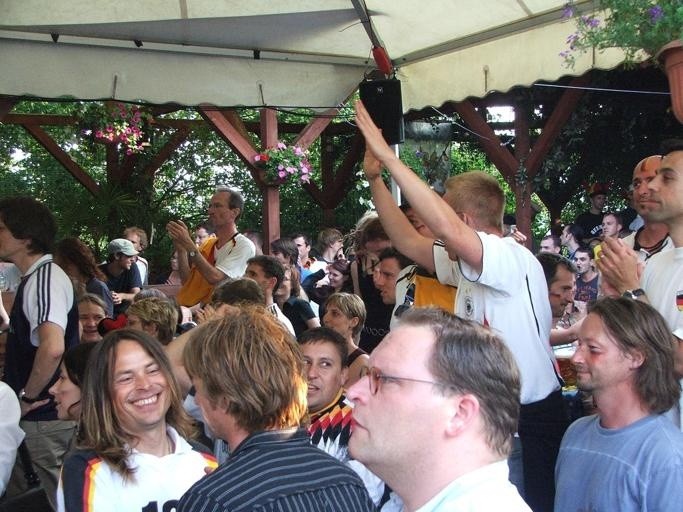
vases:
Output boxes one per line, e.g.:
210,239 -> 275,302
258,166 -> 285,186
95,130 -> 122,146
655,39 -> 683,123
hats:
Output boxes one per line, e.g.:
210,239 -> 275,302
106,238 -> 139,257
587,183 -> 608,197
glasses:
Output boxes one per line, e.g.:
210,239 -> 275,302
358,366 -> 467,395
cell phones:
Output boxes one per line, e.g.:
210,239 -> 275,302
509,225 -> 517,234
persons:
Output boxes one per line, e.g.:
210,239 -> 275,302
1,99 -> 682,511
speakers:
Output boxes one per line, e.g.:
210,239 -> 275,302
358,70 -> 406,145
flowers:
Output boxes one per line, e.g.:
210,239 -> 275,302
254,142 -> 315,186
94,101 -> 151,156
558,0 -> 683,68
